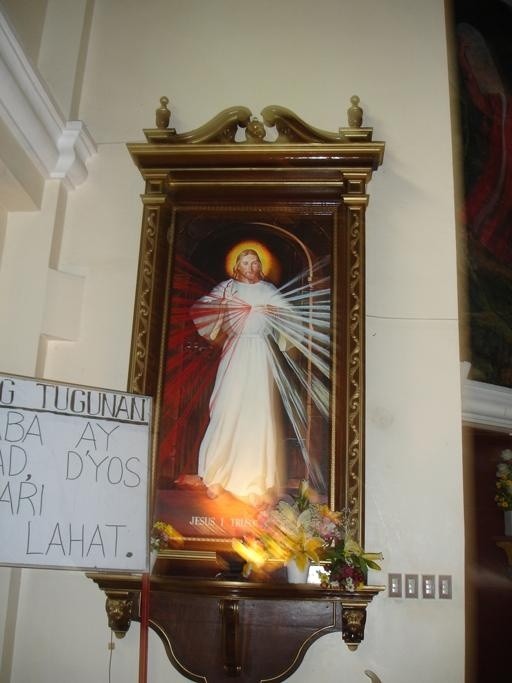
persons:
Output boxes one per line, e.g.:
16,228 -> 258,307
190,250 -> 307,501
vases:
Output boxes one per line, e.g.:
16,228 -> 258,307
149,548 -> 160,577
284,555 -> 311,584
504,510 -> 512,537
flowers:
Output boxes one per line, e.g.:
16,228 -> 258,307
229,479 -> 343,577
148,519 -> 185,550
317,543 -> 384,593
494,448 -> 512,510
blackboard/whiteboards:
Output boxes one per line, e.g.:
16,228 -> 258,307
0,373 -> 156,575
